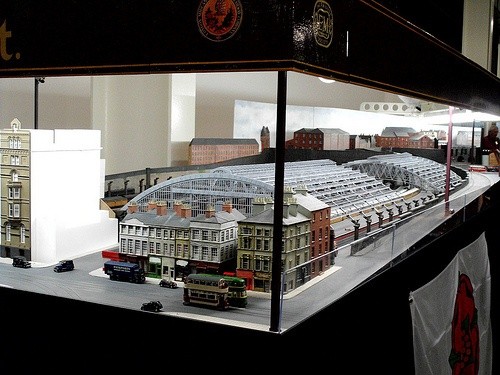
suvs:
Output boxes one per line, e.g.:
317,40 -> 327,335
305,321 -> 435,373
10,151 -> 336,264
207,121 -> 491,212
12,256 -> 31,268
54,260 -> 74,273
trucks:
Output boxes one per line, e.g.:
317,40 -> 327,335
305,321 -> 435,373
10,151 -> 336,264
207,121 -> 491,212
104,261 -> 145,283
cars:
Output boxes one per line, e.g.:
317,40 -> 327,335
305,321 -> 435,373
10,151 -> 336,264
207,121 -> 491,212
142,301 -> 163,311
159,279 -> 178,288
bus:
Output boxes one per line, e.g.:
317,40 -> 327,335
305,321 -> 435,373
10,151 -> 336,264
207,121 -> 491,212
468,165 -> 486,172
183,274 -> 247,310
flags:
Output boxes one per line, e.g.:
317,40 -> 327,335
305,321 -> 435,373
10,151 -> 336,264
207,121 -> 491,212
408,231 -> 492,374
